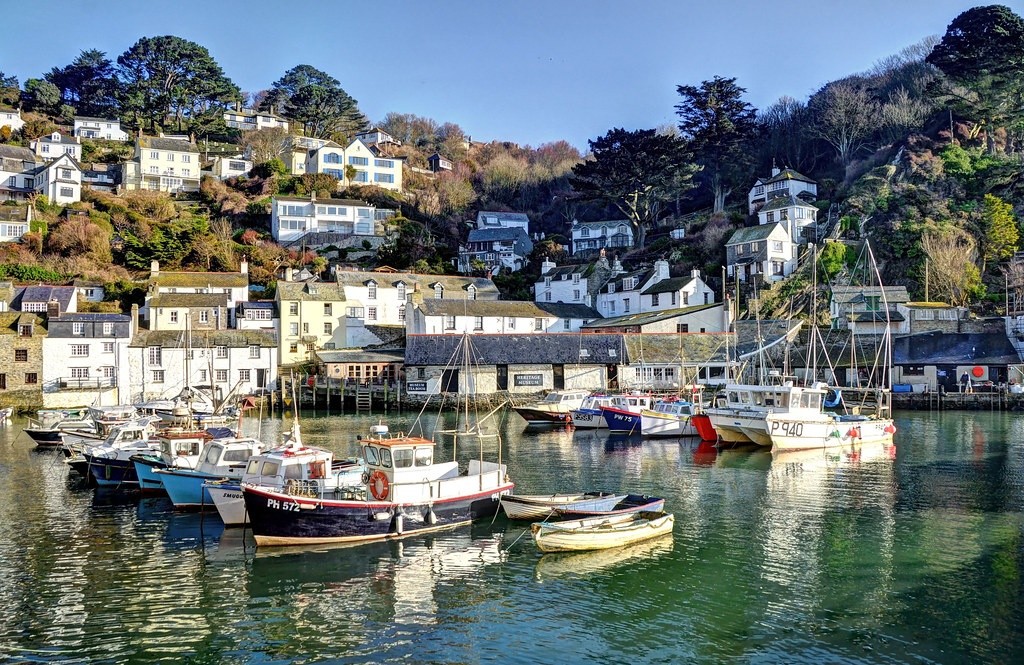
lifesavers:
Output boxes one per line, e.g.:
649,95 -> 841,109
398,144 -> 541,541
369,471 -> 389,501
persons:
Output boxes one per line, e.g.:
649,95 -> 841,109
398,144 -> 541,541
960,371 -> 972,394
997,374 -> 1006,393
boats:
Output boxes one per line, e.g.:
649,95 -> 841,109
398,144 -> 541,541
510,239 -> 898,452
497,488 -> 676,552
19,313 -> 515,548
532,531 -> 676,586
